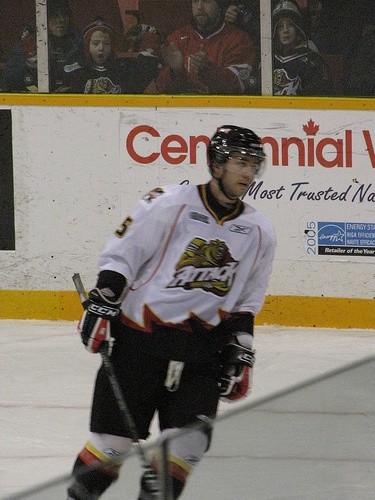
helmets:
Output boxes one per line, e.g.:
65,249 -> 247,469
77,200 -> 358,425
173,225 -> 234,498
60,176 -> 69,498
205,125 -> 266,176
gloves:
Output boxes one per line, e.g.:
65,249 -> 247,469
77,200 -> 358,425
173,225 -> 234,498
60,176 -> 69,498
222,343 -> 256,401
77,287 -> 122,357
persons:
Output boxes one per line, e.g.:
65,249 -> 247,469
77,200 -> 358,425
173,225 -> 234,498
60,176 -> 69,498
66,124 -> 277,500
0,0 -> 375,98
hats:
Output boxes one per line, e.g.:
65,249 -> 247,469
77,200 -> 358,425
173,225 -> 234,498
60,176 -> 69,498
80,17 -> 117,51
20,30 -> 40,60
273,0 -> 305,37
